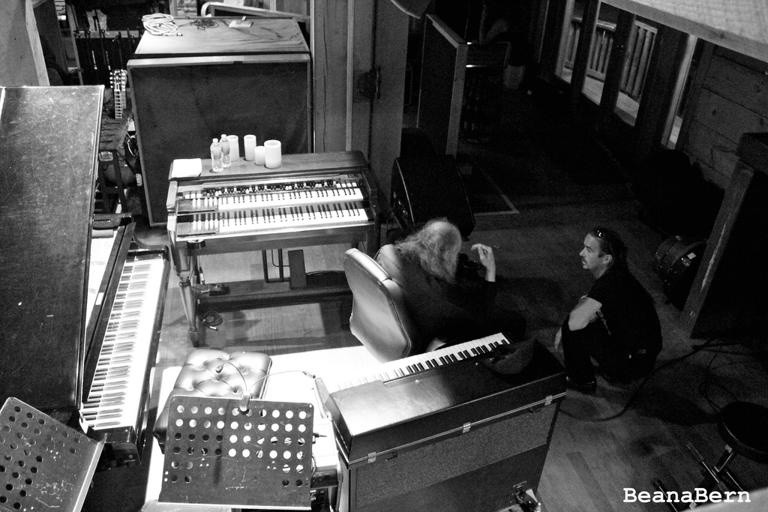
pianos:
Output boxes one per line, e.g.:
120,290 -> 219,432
322,331 -> 511,395
78,252 -> 168,430
177,170 -> 374,238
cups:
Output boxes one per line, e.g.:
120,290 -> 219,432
243,134 -> 256,161
264,140 -> 283,169
254,146 -> 265,165
228,136 -> 240,163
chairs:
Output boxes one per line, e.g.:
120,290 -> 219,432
386,153 -> 478,242
342,245 -> 446,364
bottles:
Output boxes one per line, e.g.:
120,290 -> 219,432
220,134 -> 231,168
210,138 -> 223,171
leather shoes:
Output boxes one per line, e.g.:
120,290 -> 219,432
573,379 -> 597,393
605,354 -> 651,383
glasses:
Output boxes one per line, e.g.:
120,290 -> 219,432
593,226 -> 608,239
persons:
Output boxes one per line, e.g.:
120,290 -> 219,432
554,226 -> 663,395
374,217 -> 527,343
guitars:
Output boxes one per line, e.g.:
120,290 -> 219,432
101,69 -> 138,186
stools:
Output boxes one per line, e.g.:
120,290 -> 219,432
651,399 -> 767,512
149,344 -> 274,460
